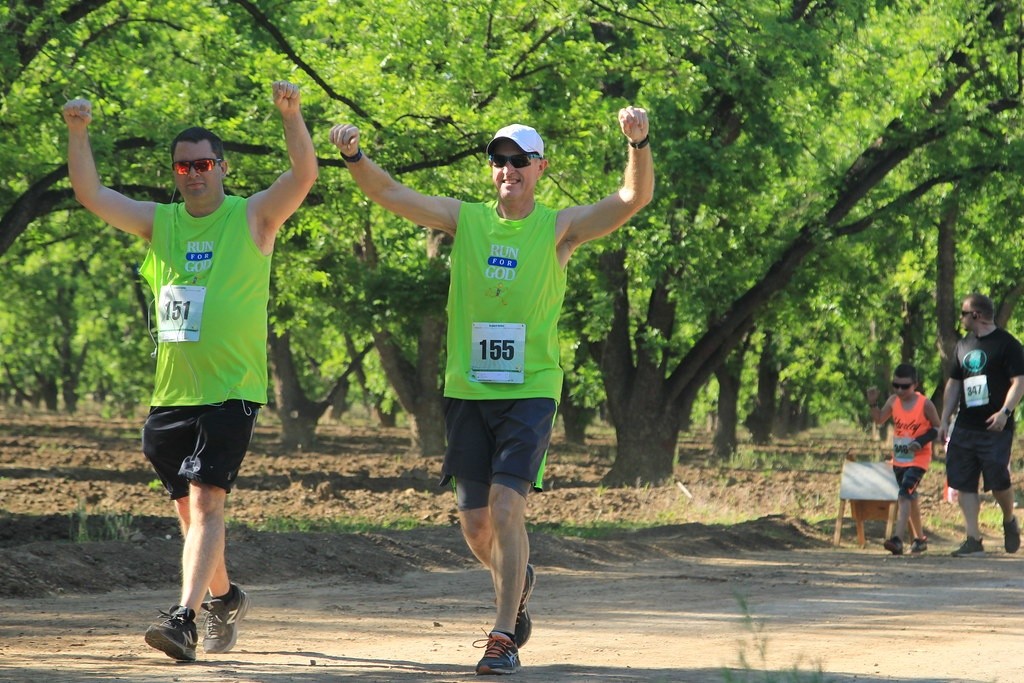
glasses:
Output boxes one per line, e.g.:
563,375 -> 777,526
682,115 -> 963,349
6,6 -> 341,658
174,157 -> 220,175
487,154 -> 540,168
961,310 -> 980,316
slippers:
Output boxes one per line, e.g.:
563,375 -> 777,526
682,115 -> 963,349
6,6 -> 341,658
890,380 -> 915,390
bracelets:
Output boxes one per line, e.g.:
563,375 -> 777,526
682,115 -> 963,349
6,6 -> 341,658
870,403 -> 878,407
629,134 -> 649,149
340,147 -> 362,162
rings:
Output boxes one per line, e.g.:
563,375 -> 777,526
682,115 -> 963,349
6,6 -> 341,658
998,426 -> 1001,430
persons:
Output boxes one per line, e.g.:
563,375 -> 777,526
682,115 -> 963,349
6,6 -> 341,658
329,105 -> 654,673
868,364 -> 941,555
65,82 -> 318,662
938,293 -> 1024,557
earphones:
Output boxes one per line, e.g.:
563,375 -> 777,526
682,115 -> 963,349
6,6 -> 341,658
973,316 -> 977,320
152,348 -> 158,358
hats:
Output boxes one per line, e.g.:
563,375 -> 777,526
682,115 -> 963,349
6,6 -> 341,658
487,122 -> 545,156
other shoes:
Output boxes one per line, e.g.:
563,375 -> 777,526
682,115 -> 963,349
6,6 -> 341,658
882,537 -> 903,555
907,535 -> 928,555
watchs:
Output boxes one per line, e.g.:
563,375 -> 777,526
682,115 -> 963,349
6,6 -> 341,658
1001,407 -> 1012,418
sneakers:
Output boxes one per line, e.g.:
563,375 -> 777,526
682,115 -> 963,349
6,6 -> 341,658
471,626 -> 524,674
951,535 -> 986,557
144,604 -> 197,664
1003,515 -> 1020,553
200,582 -> 249,654
515,563 -> 536,647
